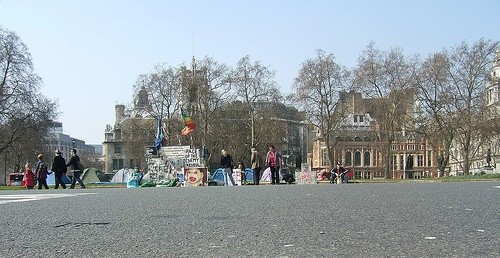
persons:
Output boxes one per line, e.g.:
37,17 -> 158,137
238,161 -> 246,185
219,149 -> 238,187
330,164 -> 348,184
33,154 -> 52,190
487,148 -> 491,167
66,149 -> 85,189
265,145 -> 282,185
138,86 -> 148,105
50,149 -> 67,189
24,163 -> 35,190
284,171 -> 296,185
251,148 -> 263,185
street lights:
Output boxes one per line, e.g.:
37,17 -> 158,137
72,139 -> 76,150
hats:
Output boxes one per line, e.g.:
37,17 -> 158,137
55,150 -> 62,154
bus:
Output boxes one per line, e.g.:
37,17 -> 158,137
9,171 -> 54,186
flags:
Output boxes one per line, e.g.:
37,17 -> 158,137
180,107 -> 196,137
152,116 -> 163,151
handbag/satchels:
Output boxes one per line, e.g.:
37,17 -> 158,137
77,160 -> 84,171
